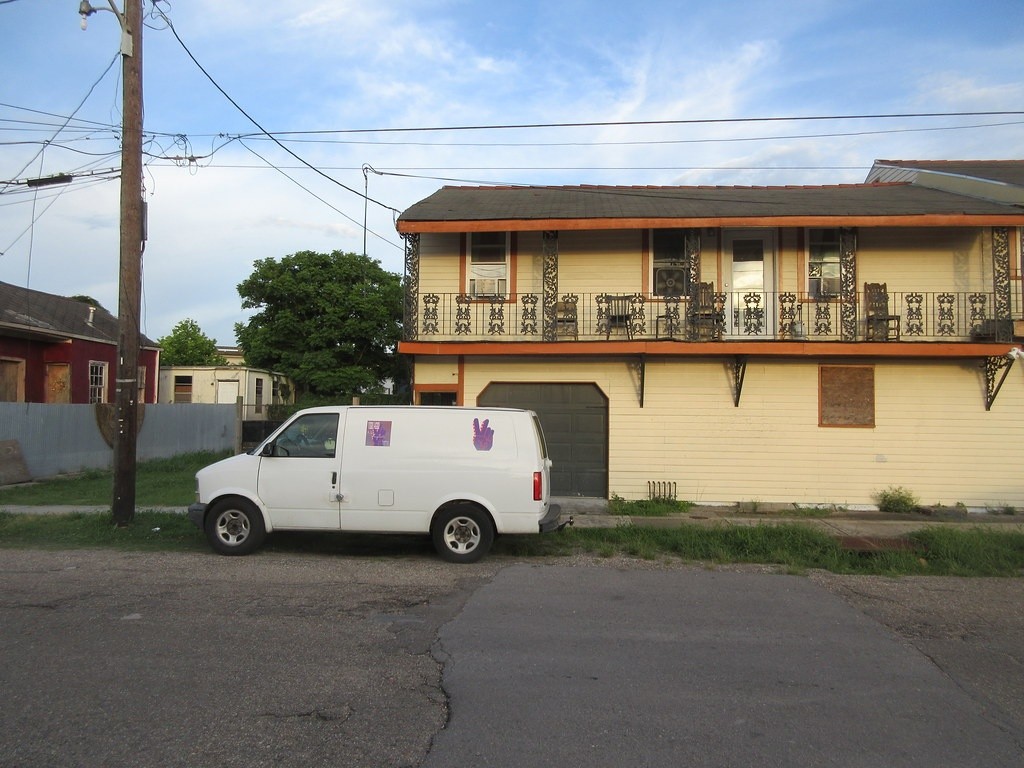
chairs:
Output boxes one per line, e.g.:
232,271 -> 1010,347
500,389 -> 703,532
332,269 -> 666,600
864,282 -> 900,341
552,302 -> 578,341
605,295 -> 633,340
689,281 -> 723,341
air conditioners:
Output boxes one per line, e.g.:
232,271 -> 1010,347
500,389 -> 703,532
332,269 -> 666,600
821,277 -> 841,296
475,278 -> 498,297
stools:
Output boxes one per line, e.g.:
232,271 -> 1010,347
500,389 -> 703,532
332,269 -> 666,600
656,315 -> 677,339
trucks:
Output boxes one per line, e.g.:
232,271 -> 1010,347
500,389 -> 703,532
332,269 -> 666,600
186,405 -> 553,565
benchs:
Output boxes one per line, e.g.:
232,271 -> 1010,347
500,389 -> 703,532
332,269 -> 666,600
969,319 -> 1016,341
